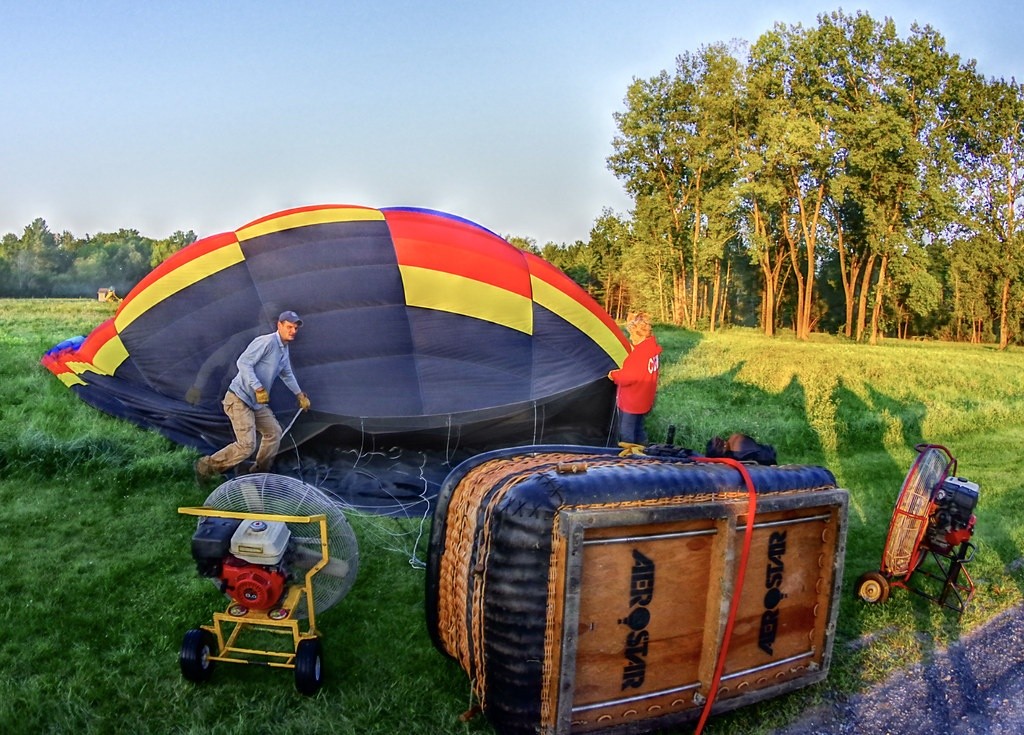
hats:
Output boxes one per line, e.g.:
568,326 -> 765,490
279,312 -> 303,328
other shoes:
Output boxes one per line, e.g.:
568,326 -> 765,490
198,458 -> 211,478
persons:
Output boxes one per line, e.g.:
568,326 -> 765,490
608,311 -> 663,446
198,311 -> 311,480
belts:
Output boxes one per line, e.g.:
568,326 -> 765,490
228,387 -> 236,394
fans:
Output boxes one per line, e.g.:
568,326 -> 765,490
176,472 -> 359,697
857,444 -> 984,623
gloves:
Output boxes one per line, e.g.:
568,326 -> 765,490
256,388 -> 268,403
298,395 -> 311,413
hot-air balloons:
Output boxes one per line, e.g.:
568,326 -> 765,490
41,204 -> 850,735
